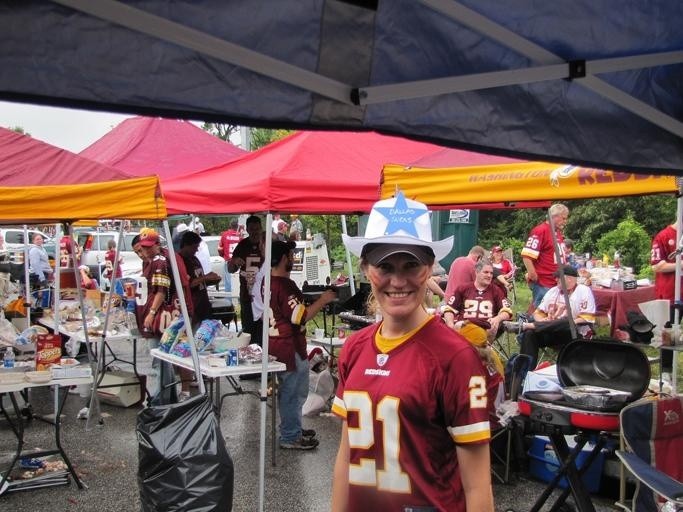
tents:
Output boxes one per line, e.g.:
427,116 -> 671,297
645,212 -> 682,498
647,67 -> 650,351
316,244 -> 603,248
162,121 -> 582,343
1,125 -> 211,402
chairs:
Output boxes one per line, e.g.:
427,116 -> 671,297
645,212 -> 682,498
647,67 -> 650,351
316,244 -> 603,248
484,249 -> 518,304
615,396 -> 682,512
488,351 -> 532,489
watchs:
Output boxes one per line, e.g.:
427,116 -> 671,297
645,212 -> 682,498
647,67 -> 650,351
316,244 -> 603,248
149,307 -> 157,316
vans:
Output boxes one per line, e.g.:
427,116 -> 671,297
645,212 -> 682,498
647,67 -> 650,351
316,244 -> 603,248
0,229 -> 53,251
75,232 -> 165,266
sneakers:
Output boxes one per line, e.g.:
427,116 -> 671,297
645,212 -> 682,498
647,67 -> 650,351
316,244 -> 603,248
279,437 -> 319,450
178,391 -> 191,403
502,317 -> 524,335
302,428 -> 316,438
239,374 -> 259,380
258,385 -> 279,396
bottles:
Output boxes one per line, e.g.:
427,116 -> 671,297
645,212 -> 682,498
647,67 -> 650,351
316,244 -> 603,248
4,347 -> 15,368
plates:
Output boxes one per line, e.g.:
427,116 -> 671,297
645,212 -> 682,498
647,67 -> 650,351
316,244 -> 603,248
61,358 -> 80,366
25,375 -> 54,382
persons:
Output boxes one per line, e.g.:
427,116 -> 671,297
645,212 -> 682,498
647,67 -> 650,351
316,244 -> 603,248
277,222 -> 287,242
439,245 -> 484,306
79,265 -> 96,290
176,221 -> 187,232
562,239 -> 578,268
329,193 -> 495,512
60,227 -> 83,268
218,220 -> 243,303
189,216 -> 205,233
489,245 -> 514,292
288,214 -> 304,241
28,233 -> 53,290
515,203 -> 569,347
443,261 -> 513,345
260,240 -> 337,451
503,264 -> 597,371
134,228 -> 178,408
250,230 -> 284,397
131,234 -> 195,403
176,231 -> 222,387
227,216 -> 264,381
272,214 -> 287,234
650,220 -> 683,384
105,239 -> 122,285
426,257 -> 448,304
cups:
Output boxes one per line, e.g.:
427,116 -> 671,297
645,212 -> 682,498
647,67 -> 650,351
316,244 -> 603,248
313,329 -> 324,339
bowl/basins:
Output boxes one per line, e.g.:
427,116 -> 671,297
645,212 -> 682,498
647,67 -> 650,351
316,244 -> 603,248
26,371 -> 50,379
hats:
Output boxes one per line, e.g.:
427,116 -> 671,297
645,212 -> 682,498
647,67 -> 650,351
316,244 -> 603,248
277,222 -> 290,230
341,191 -> 455,262
492,246 -> 504,253
135,228 -> 161,248
271,240 -> 296,261
553,264 -> 580,277
360,243 -> 435,267
195,217 -> 200,223
